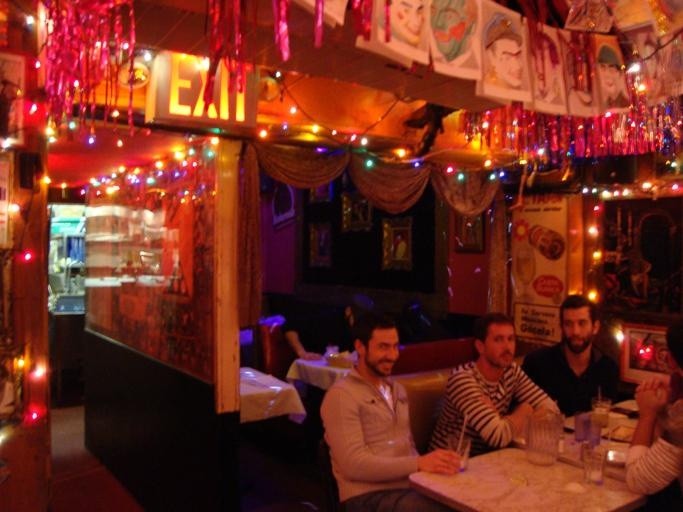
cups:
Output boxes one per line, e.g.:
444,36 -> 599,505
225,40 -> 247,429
576,413 -> 591,444
524,409 -> 563,465
584,421 -> 602,446
579,441 -> 607,486
445,433 -> 473,470
592,398 -> 613,430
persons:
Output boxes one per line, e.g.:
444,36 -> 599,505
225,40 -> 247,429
519,293 -> 626,417
428,314 -> 560,464
320,313 -> 463,511
254,287 -> 430,372
623,320 -> 683,495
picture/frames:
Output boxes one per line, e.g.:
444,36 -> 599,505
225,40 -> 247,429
619,322 -> 673,386
454,212 -> 486,253
308,183 -> 413,271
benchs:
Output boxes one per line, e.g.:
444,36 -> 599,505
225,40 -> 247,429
261,326 -> 524,374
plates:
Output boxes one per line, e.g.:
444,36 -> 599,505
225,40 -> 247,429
600,442 -> 631,466
614,398 -> 641,414
